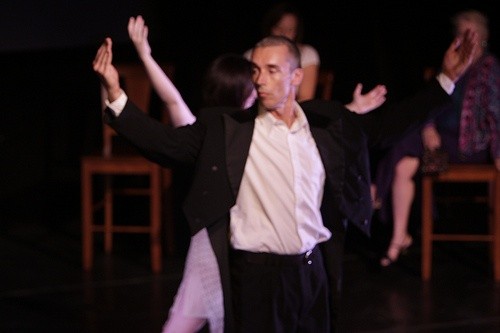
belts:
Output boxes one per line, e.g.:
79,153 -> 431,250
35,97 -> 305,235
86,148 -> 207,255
229,244 -> 321,268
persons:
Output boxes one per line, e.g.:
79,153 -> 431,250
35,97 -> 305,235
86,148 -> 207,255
242,12 -> 320,102
379,9 -> 500,269
92,27 -> 479,333
126,14 -> 387,333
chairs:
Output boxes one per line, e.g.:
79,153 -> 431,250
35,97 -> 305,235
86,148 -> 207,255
79,65 -> 174,274
421,164 -> 500,283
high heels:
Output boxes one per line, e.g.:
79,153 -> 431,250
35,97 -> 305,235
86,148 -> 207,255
380,235 -> 413,268
